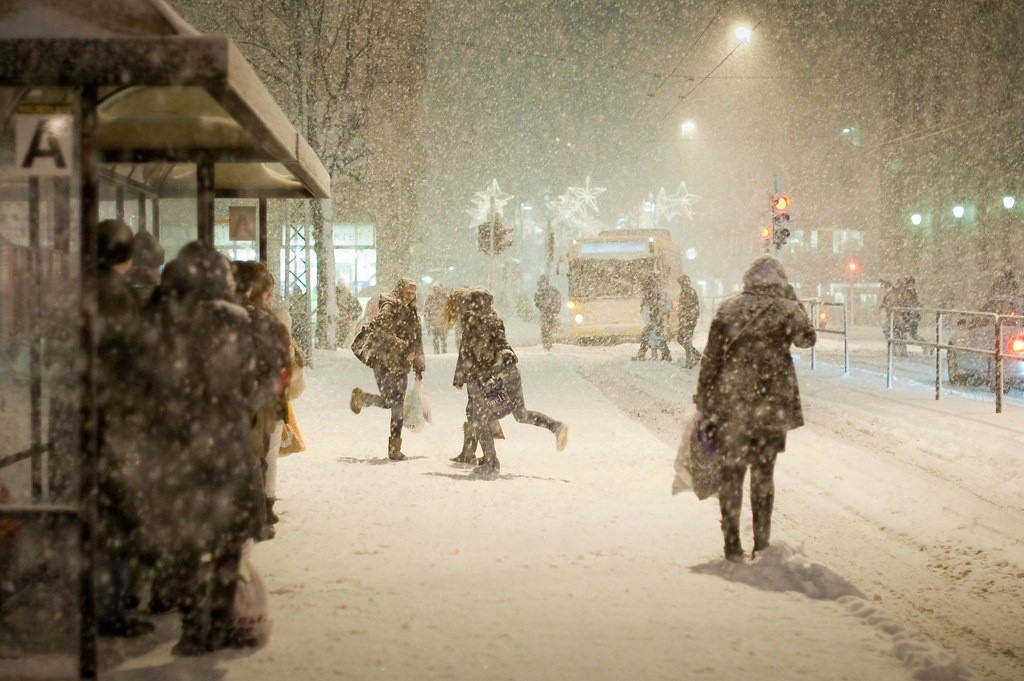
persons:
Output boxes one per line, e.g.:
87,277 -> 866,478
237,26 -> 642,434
691,253 -> 817,563
53,218 -> 300,658
532,275 -> 562,351
334,277 -> 362,347
364,283 -> 385,327
983,265 -> 1020,327
423,282 -> 569,477
630,274 -> 702,369
349,277 -> 425,460
875,275 -> 936,357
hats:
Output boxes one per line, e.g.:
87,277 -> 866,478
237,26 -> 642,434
132,231 -> 164,267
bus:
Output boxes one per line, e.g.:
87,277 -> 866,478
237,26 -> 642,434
556,229 -> 684,346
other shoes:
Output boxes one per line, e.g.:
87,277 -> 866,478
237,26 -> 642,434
478,437 -> 496,465
557,426 -> 567,452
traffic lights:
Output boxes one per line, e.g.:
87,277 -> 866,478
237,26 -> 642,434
759,226 -> 771,247
774,196 -> 791,244
494,222 -> 514,253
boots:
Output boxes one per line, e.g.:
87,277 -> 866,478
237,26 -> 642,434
171,579 -> 256,655
751,511 -> 772,560
389,437 -> 405,459
449,422 -> 480,463
719,515 -> 743,561
350,388 -> 370,415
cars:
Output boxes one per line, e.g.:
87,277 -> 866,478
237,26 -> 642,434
947,295 -> 1024,395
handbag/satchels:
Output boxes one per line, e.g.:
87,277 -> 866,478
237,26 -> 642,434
352,325 -> 386,368
670,410 -> 723,501
402,377 -> 432,431
280,398 -> 306,455
